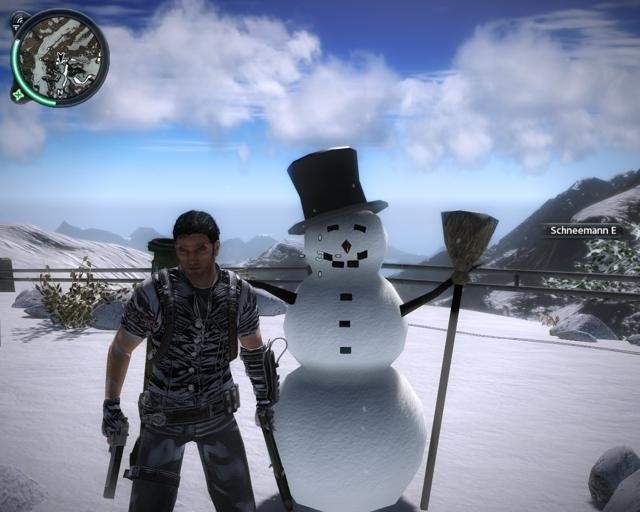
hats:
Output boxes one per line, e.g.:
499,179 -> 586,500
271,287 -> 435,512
286,143 -> 388,234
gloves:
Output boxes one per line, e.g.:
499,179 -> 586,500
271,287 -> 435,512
101,398 -> 127,439
255,405 -> 277,433
